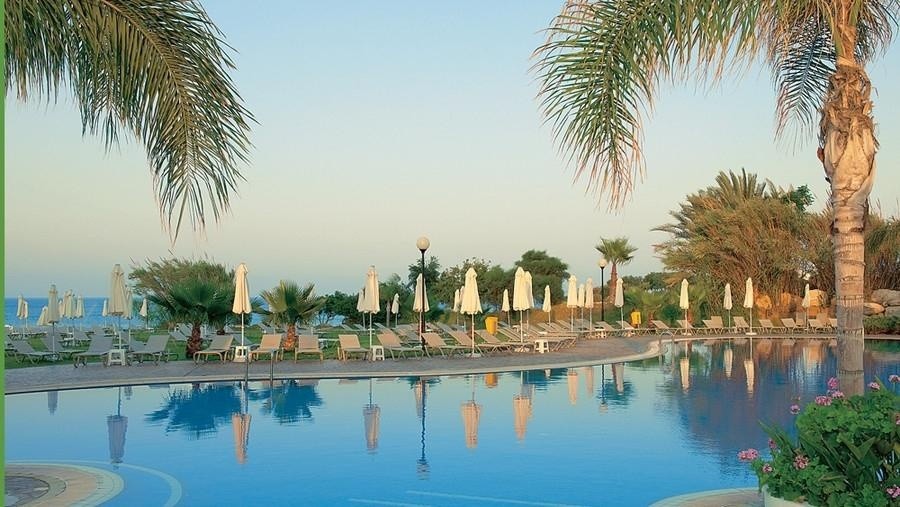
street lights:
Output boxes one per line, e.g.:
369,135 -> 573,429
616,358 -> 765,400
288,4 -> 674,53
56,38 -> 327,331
416,378 -> 431,482
416,236 -> 431,358
597,258 -> 608,330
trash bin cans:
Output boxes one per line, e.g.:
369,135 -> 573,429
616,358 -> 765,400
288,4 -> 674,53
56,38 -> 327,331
630,310 -> 642,325
484,315 -> 498,335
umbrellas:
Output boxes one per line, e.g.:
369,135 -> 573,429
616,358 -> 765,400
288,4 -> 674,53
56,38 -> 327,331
16,284 -> 147,357
48,392 -> 58,416
585,367 -> 594,399
514,371 -> 530,442
724,339 -> 734,379
414,377 -> 429,420
107,388 -> 128,470
125,385 -> 133,402
679,279 -> 690,332
743,277 -> 754,333
363,378 -> 381,456
680,342 -> 690,392
724,283 -> 732,328
614,278 -> 625,329
357,265 -> 595,353
232,381 -> 252,465
567,368 -> 577,406
743,338 -> 755,397
108,263 -> 129,350
232,263 -> 252,346
461,375 -> 481,448
612,363 -> 625,395
801,283 -> 811,328
